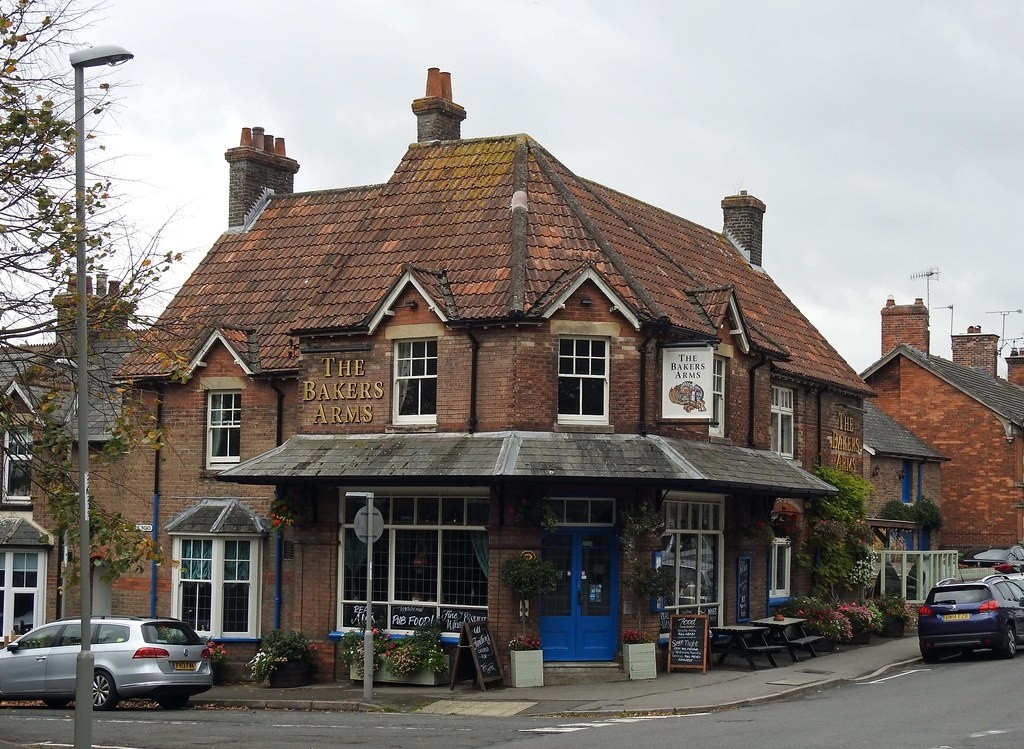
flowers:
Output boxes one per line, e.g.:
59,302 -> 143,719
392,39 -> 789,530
617,501 -> 665,562
244,625 -> 319,683
743,514 -> 828,546
207,637 -> 227,662
268,499 -> 300,536
506,496 -> 558,534
794,596 -> 920,642
341,614 -> 448,678
622,628 -> 653,644
507,634 -> 541,650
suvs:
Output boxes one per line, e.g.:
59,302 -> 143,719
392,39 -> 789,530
0,615 -> 215,709
917,573 -> 1024,659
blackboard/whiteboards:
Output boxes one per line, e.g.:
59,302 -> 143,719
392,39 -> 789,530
451,620 -> 504,682
668,614 -> 712,668
388,602 -> 438,631
738,557 -> 751,622
661,609 -> 676,634
341,601 -> 388,630
679,606 -> 698,613
700,607 -> 718,626
437,604 -> 488,633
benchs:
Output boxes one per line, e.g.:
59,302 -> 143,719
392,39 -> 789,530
789,635 -> 827,645
746,645 -> 786,653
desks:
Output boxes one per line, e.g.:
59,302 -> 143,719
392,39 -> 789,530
710,625 -> 778,670
750,616 -> 819,662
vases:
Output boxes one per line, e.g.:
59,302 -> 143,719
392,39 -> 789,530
639,531 -> 658,547
510,649 -> 544,688
350,652 -> 451,685
622,640 -> 657,681
270,660 -> 313,688
212,662 -> 225,686
512,512 -> 536,531
805,618 -> 908,649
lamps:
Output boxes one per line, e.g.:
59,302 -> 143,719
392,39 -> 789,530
872,465 -> 880,477
896,467 -> 905,479
405,299 -> 418,308
581,297 -> 593,305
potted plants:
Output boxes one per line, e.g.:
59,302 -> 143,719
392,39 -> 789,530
774,606 -> 784,621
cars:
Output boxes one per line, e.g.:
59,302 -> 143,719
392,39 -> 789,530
661,533 -> 714,562
958,545 -> 1024,574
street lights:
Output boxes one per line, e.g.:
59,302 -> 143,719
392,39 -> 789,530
344,490 -> 377,701
73,46 -> 136,749
